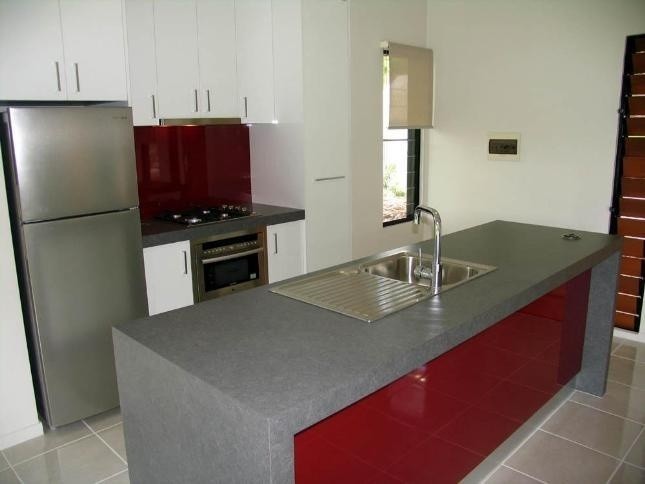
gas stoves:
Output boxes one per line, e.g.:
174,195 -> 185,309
163,202 -> 253,225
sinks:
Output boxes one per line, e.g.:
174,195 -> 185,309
360,254 -> 480,287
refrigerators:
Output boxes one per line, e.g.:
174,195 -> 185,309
0,102 -> 151,429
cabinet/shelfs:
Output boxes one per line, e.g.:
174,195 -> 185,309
0,0 -> 130,102
266,216 -> 309,284
141,239 -> 195,316
154,0 -> 245,128
124,1 -> 158,126
236,0 -> 305,125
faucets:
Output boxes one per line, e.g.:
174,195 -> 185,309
411,204 -> 444,296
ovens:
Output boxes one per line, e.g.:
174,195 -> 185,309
192,228 -> 268,306
